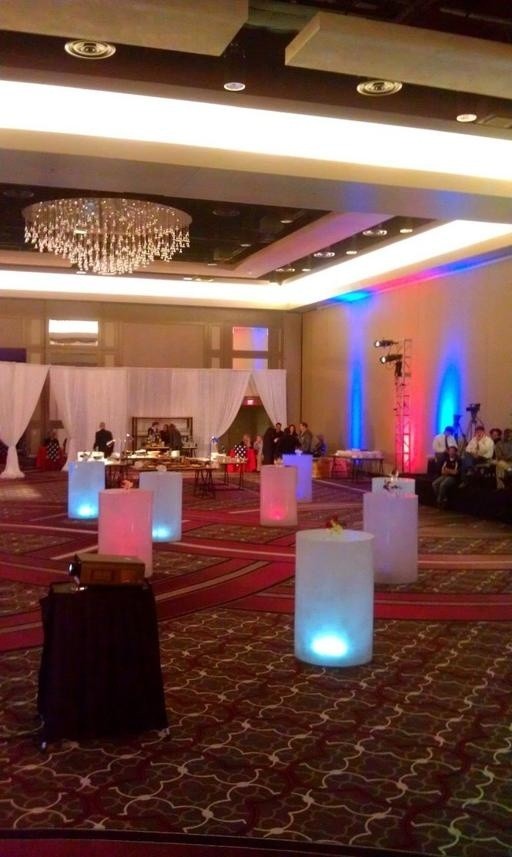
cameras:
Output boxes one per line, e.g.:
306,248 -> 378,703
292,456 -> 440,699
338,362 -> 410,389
466,403 -> 480,412
454,414 -> 461,419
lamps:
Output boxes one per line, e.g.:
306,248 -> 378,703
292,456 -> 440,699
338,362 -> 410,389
373,339 -> 402,363
212,436 -> 218,446
106,433 -> 134,465
21,197 -> 192,276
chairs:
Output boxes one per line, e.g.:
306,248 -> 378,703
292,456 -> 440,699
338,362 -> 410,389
35,447 -> 67,471
224,448 -> 256,473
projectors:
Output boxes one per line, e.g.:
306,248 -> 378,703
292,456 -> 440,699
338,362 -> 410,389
67,553 -> 146,588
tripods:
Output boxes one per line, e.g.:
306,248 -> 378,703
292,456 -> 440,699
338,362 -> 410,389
454,419 -> 467,446
468,412 -> 477,441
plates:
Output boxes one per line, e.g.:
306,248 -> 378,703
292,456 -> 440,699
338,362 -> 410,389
335,446 -> 384,458
89,446 -> 238,465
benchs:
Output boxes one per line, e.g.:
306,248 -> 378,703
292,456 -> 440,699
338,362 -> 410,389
415,459 -> 512,524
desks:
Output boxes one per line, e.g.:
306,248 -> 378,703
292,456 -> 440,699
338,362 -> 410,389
38,581 -> 170,753
330,455 -> 385,483
77,447 -> 247,500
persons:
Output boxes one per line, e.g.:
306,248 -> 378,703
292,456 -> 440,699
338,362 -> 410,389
90,421 -> 116,458
428,426 -> 511,512
239,422 -> 327,474
158,424 -> 171,452
168,424 -> 184,455
146,421 -> 159,441
40,427 -> 61,471
